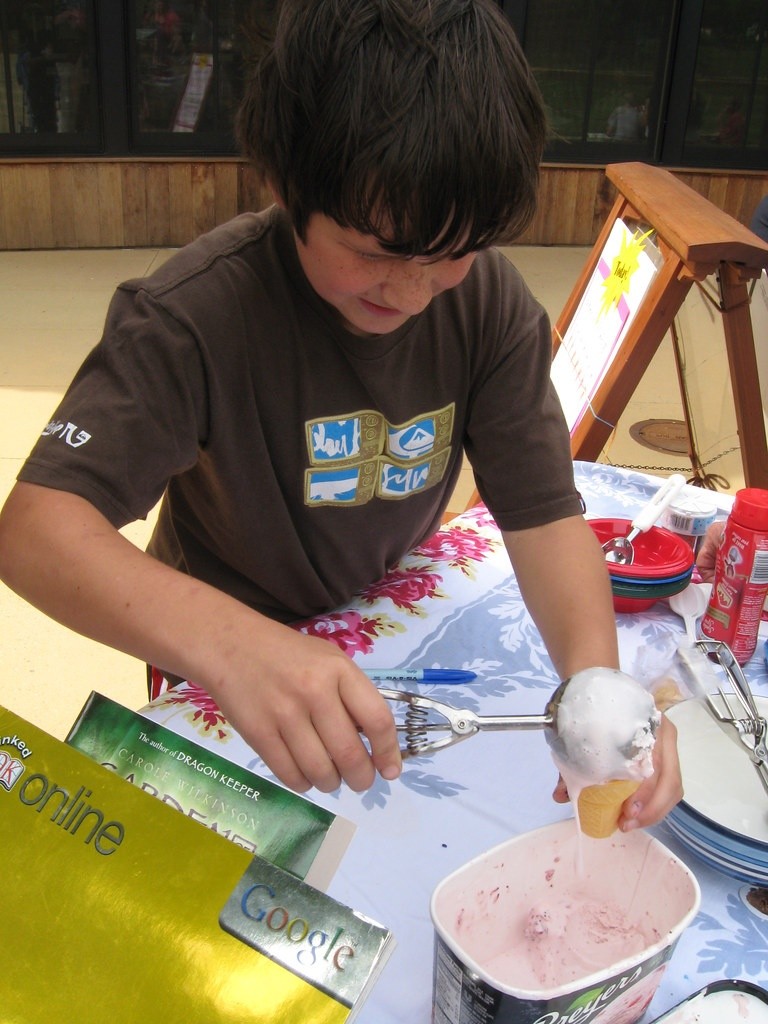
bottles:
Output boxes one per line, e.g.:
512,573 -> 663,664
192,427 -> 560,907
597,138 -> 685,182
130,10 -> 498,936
696,485 -> 768,669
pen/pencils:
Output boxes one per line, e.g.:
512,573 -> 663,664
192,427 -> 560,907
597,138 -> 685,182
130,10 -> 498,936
361,668 -> 480,685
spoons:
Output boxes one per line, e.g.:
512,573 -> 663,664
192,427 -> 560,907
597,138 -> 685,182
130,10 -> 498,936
600,474 -> 689,568
668,583 -> 706,645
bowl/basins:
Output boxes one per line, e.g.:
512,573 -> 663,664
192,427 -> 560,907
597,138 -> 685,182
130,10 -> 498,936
585,517 -> 695,613
658,691 -> 767,888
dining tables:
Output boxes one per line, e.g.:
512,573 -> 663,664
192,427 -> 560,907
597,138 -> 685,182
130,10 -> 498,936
136,458 -> 767,1024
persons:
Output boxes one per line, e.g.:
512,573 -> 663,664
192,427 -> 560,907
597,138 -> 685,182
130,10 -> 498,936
0,0 -> 686,839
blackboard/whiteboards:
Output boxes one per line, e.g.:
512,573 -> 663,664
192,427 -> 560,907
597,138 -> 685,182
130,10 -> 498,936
544,160 -> 767,491
